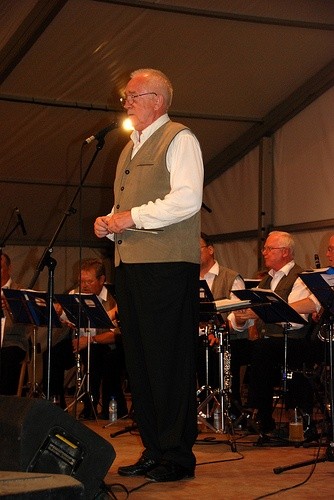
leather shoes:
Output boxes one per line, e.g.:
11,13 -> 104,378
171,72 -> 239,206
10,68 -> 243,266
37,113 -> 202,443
118,449 -> 160,476
147,458 -> 195,482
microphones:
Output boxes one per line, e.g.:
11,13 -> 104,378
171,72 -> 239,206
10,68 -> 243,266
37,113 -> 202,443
83,120 -> 121,145
16,206 -> 27,235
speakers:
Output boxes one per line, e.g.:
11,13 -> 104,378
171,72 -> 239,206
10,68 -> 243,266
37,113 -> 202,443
0,395 -> 116,490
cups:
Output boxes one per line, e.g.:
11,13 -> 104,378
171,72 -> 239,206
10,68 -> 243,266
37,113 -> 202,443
290,408 -> 310,441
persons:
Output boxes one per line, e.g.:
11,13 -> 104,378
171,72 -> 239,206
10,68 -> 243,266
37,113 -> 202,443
94,69 -> 204,482
325,232 -> 334,270
114,335 -> 134,419
0,254 -> 28,395
232,230 -> 323,440
199,232 -> 246,422
41,261 -> 118,419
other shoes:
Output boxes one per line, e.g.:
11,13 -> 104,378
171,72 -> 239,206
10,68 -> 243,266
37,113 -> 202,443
100,407 -> 110,419
79,406 -> 97,420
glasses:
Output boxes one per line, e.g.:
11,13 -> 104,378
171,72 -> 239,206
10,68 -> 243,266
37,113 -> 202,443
261,246 -> 282,252
120,92 -> 157,106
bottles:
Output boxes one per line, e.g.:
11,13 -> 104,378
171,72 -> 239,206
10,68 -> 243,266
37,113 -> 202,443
109,397 -> 118,423
214,405 -> 222,430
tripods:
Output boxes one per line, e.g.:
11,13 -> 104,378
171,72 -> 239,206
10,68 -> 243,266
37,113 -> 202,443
29,139 -> 137,439
196,321 -> 334,474
